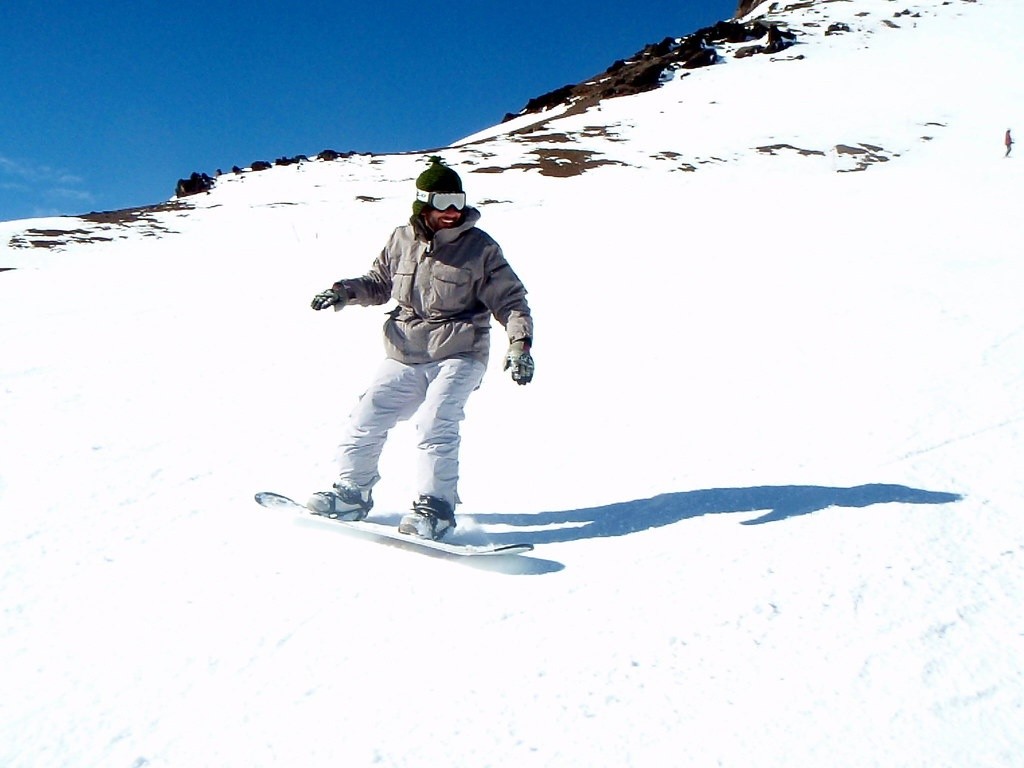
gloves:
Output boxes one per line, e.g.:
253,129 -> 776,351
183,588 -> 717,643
503,340 -> 535,385
311,289 -> 339,310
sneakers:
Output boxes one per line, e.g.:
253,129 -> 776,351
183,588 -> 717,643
310,482 -> 373,520
400,494 -> 455,539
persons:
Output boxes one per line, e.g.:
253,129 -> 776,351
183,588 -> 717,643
308,157 -> 535,539
1004,128 -> 1011,156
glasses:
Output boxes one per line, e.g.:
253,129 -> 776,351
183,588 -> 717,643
429,192 -> 466,211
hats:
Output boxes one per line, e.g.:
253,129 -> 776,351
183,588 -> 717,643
410,156 -> 462,237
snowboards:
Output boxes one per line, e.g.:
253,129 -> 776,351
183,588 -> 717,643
255,490 -> 535,557
